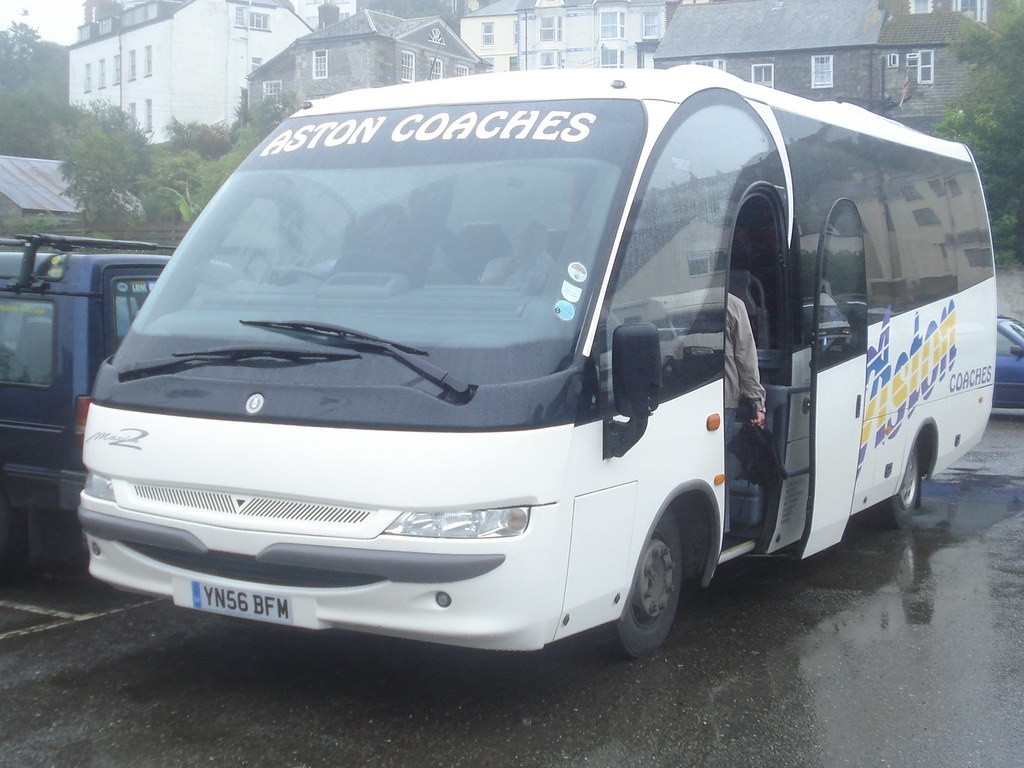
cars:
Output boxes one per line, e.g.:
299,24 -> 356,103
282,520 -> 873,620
991,316 -> 1024,413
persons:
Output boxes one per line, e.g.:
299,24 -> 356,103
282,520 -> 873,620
482,222 -> 556,295
723,292 -> 766,546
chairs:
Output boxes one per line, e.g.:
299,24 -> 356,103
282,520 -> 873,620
16,307 -> 52,384
728,270 -> 769,349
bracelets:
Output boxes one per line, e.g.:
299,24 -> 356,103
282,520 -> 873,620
756,407 -> 766,413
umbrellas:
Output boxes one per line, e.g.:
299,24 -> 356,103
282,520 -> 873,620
727,414 -> 789,490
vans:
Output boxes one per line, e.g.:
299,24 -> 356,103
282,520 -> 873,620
82,66 -> 999,667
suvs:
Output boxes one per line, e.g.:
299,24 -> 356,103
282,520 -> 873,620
1,234 -> 237,542
